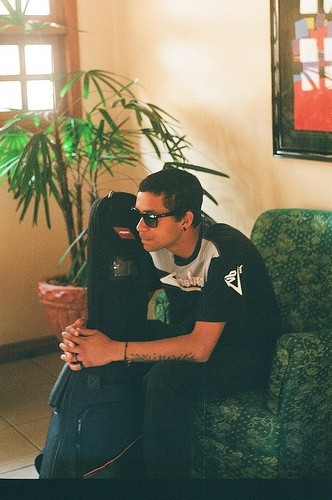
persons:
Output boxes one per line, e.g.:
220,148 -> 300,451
34,170 -> 282,481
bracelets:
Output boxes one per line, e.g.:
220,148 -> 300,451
123,339 -> 130,365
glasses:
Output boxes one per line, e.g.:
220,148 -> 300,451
130,207 -> 185,227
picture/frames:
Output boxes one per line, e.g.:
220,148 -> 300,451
269,0 -> 332,162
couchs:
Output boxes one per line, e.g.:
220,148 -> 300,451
183,209 -> 332,482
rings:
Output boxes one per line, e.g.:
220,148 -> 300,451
79,363 -> 83,371
73,353 -> 78,363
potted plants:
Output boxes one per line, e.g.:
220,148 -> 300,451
0,0 -> 231,342
